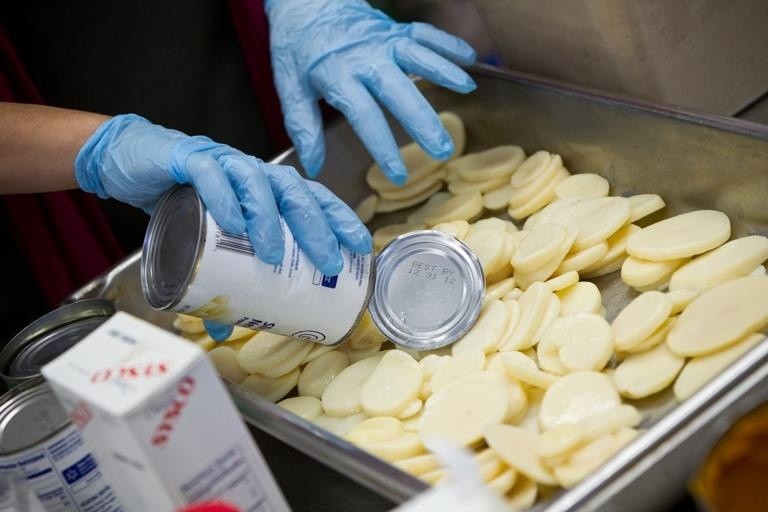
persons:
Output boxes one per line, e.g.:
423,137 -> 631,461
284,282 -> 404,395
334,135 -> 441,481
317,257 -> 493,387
0,1 -> 479,347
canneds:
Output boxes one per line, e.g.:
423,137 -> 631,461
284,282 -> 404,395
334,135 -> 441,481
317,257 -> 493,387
0,373 -> 126,512
0,296 -> 118,389
140,183 -> 485,350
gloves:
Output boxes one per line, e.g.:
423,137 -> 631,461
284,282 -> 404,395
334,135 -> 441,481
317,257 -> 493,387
72,109 -> 375,274
260,0 -> 484,189
